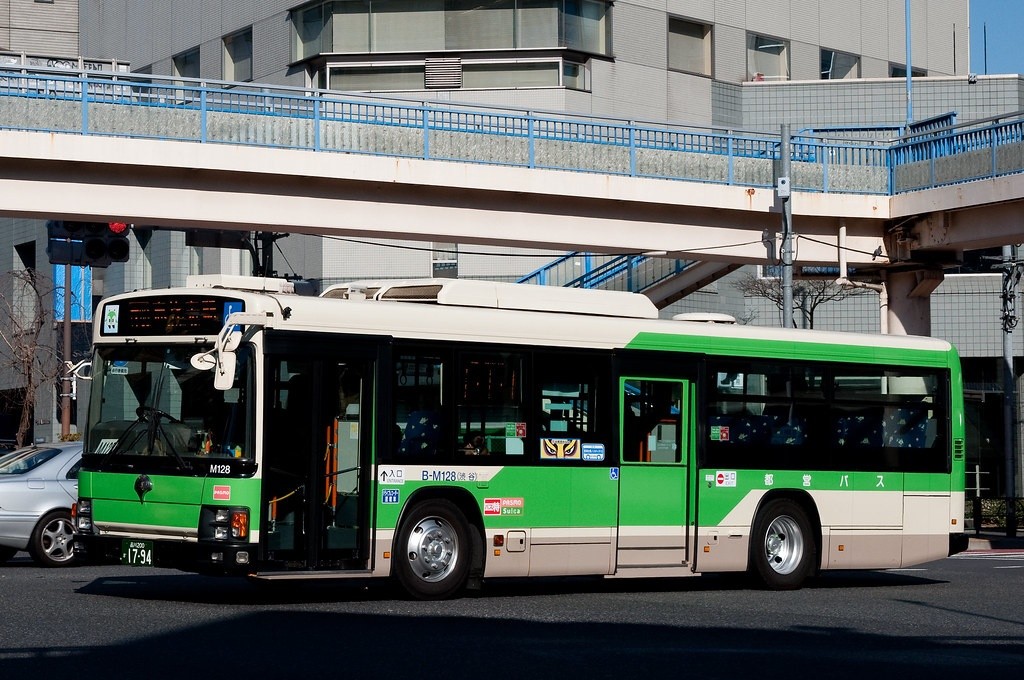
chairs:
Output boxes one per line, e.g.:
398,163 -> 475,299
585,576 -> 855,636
707,415 -> 809,447
399,410 -> 445,456
835,401 -> 927,446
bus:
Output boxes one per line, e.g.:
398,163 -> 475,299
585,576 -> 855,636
74,275 -> 971,585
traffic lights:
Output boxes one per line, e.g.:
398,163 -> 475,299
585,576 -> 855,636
84,221 -> 129,262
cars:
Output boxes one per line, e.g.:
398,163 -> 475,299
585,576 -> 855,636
0,440 -> 84,565
